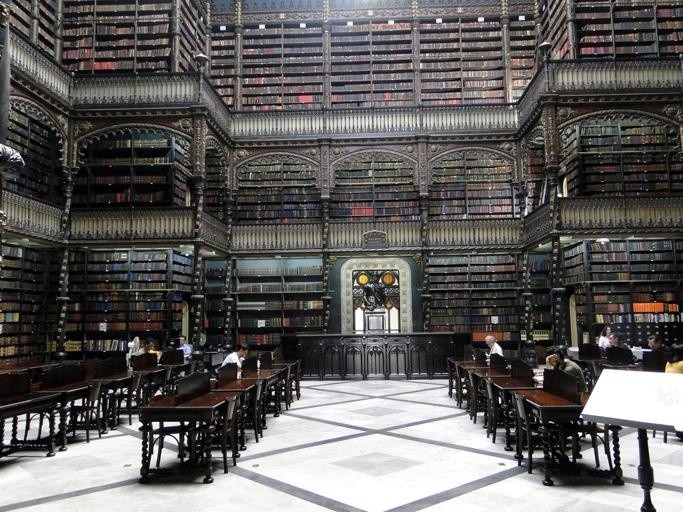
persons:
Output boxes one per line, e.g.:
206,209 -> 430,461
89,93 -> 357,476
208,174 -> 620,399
648,334 -> 671,353
599,325 -> 612,349
174,335 -> 193,377
544,345 -> 589,409
135,339 -> 162,363
484,335 -> 504,361
220,343 -> 249,376
609,333 -> 624,349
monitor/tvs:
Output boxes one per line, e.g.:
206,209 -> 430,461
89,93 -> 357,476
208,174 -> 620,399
165,338 -> 180,351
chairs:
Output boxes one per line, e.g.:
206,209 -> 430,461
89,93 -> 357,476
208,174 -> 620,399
137,350 -> 300,489
444,345 -> 682,488
1,351 -> 223,457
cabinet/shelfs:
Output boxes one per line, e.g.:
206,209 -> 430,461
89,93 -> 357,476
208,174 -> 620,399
0,0 -> 682,351
